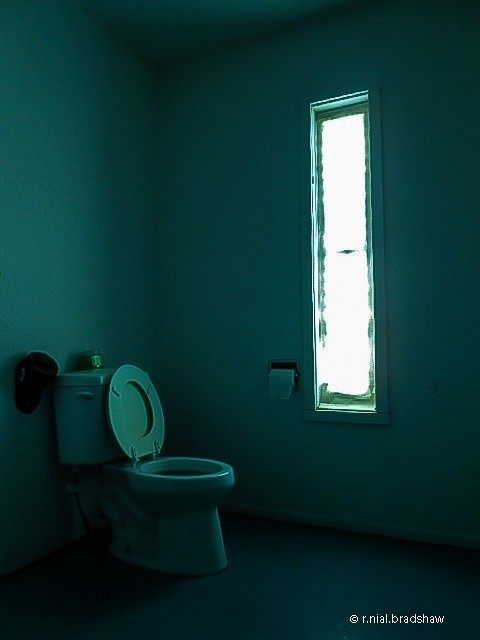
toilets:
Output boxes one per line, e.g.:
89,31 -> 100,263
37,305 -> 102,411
55,364 -> 234,574
